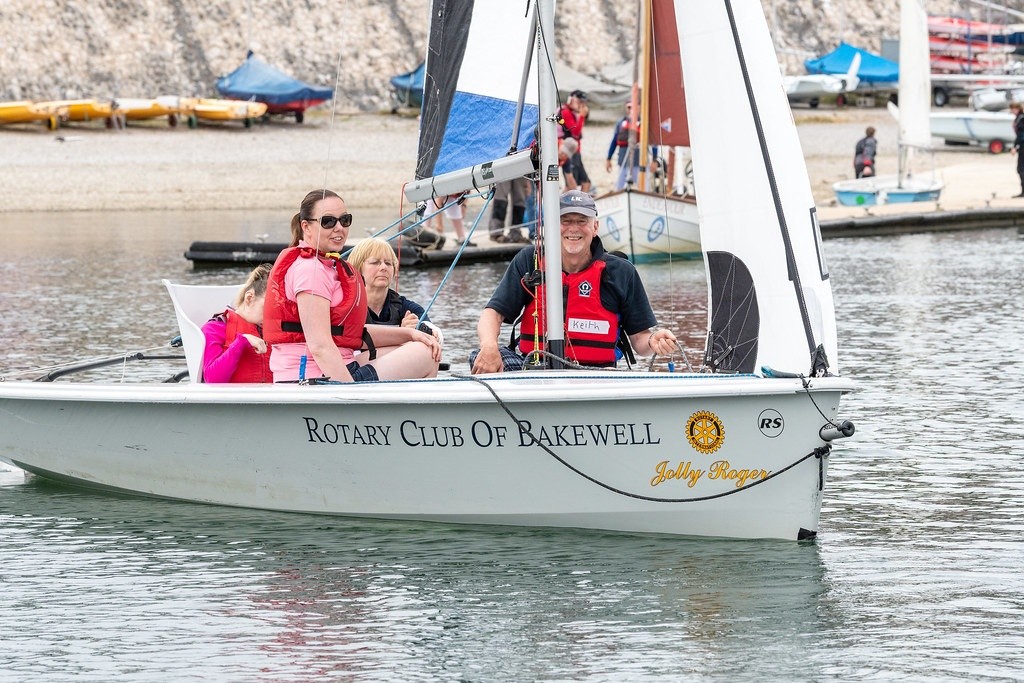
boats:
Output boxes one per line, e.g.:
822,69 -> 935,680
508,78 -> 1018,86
832,172 -> 944,208
388,59 -> 427,108
215,50 -> 333,111
926,14 -> 1024,95
775,53 -> 862,103
881,98 -> 1019,141
804,39 -> 903,85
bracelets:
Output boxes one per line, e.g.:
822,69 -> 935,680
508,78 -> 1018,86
648,331 -> 653,347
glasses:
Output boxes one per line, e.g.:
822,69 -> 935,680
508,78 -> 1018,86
302,213 -> 352,230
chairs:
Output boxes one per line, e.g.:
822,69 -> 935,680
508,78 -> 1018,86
163,279 -> 251,384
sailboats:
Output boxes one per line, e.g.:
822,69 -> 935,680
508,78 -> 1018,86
2,0 -> 858,547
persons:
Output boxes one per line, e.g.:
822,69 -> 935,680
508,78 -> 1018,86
200,263 -> 274,382
262,189 -> 443,382
853,126 -> 877,179
1008,103 -> 1024,198
348,238 -> 443,347
469,190 -> 678,376
421,89 -> 658,246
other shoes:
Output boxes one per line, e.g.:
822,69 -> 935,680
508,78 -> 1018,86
490,234 -> 512,243
508,230 -> 532,245
456,239 -> 476,247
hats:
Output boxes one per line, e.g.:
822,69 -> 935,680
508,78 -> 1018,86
626,102 -> 632,107
560,190 -> 598,216
571,90 -> 590,100
560,137 -> 578,158
